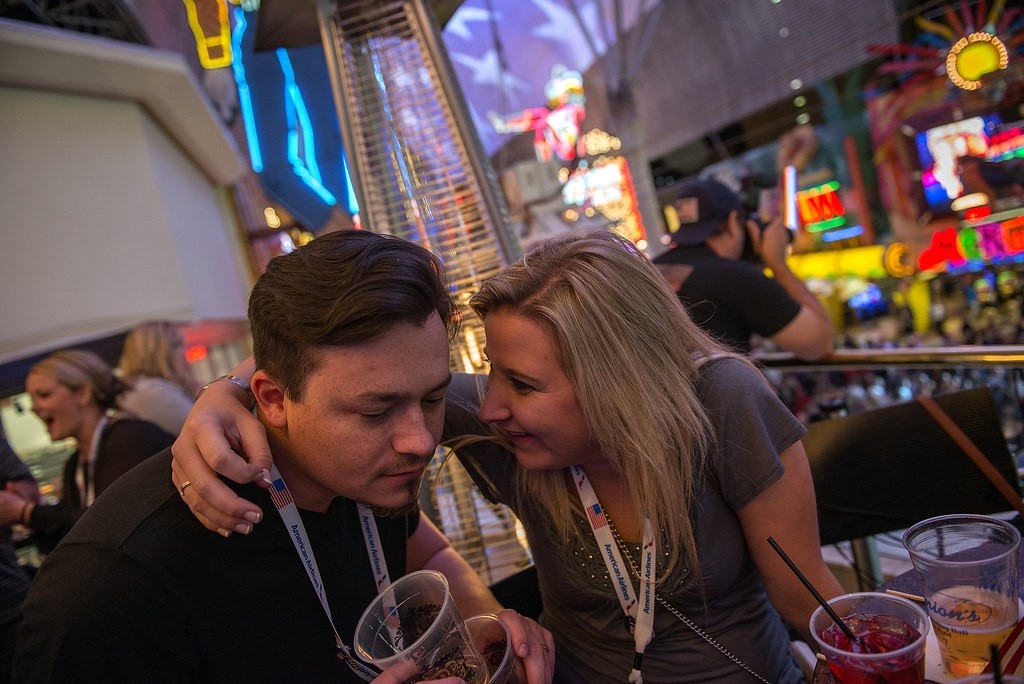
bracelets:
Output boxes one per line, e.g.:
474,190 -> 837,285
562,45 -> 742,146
196,374 -> 256,409
21,501 -> 32,521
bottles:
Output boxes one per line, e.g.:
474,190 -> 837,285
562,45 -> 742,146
972,266 -> 1024,334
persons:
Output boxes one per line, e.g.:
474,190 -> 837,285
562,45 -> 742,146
647,174 -> 836,360
0,423 -> 39,617
0,348 -> 175,543
0,230 -> 557,684
172,230 -> 853,684
113,320 -> 202,437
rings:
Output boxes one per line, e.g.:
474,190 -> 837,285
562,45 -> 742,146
179,480 -> 192,496
540,644 -> 549,652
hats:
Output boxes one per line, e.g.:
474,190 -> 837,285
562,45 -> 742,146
672,178 -> 741,243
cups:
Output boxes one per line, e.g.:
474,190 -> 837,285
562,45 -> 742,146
35,479 -> 60,507
354,569 -> 528,684
810,514 -> 1021,684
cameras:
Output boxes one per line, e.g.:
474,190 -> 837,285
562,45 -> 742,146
743,222 -> 793,259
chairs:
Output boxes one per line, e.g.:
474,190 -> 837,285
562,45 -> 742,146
799,384 -> 1024,549
